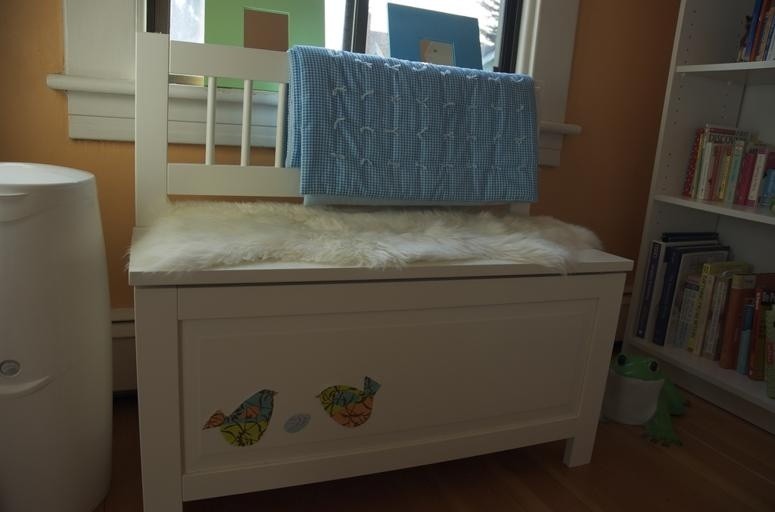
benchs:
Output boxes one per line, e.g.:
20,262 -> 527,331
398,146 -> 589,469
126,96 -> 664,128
128,36 -> 636,511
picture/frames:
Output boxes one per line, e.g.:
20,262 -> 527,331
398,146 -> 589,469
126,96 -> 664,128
204,0 -> 324,91
389,3 -> 484,70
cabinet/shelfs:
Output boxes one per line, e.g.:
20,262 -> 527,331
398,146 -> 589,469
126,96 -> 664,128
622,0 -> 775,432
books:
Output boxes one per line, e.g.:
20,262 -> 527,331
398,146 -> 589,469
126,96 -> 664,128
737,0 -> 775,62
679,123 -> 775,212
632,227 -> 775,403
